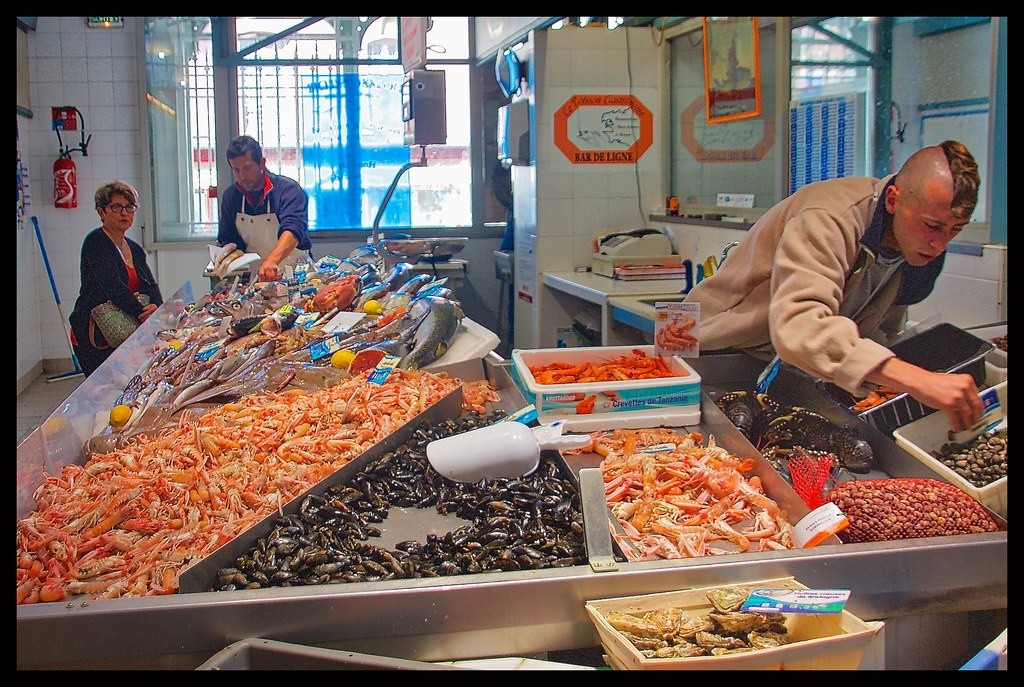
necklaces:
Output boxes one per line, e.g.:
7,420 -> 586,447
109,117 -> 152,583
116,241 -> 130,262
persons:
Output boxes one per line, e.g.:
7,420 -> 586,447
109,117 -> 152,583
681,141 -> 986,429
68,182 -> 163,378
217,136 -> 316,281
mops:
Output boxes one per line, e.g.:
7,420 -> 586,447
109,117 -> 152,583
28,212 -> 87,384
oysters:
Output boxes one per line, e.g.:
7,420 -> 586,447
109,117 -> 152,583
605,586 -> 796,659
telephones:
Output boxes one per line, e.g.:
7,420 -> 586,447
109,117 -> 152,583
599,228 -> 674,259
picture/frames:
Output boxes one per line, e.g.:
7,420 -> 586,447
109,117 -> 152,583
700,14 -> 763,124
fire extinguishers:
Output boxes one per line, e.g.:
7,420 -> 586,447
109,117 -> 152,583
51,148 -> 79,210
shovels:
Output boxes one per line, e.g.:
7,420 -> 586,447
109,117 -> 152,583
426,418 -> 592,486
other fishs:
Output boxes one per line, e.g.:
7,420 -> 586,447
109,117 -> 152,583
213,243 -> 237,270
89,252 -> 467,455
202,249 -> 244,283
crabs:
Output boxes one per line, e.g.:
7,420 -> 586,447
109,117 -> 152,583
847,385 -> 904,412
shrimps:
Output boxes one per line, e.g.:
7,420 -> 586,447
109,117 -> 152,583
569,393 -> 585,401
576,395 -> 597,414
17,367 -> 502,605
528,347 -> 679,385
656,314 -> 699,352
558,427 -> 796,563
602,391 -> 616,397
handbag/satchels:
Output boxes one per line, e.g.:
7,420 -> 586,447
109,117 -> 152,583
88,293 -> 150,350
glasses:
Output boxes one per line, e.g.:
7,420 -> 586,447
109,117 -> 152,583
102,203 -> 137,213
